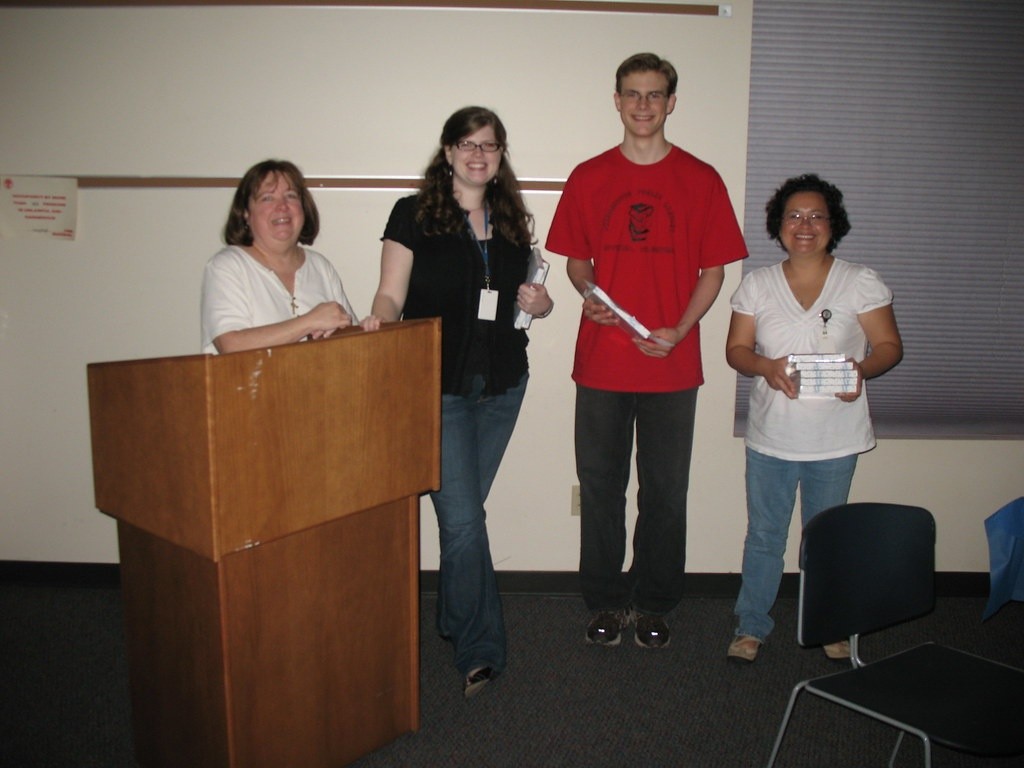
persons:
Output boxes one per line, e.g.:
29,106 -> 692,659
545,53 -> 752,653
728,173 -> 904,659
356,107 -> 556,697
200,158 -> 360,356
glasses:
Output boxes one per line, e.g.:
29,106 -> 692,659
780,209 -> 830,226
452,140 -> 501,153
619,89 -> 667,105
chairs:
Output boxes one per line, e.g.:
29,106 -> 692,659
764,501 -> 1023,768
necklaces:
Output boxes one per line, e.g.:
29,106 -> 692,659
787,273 -> 813,305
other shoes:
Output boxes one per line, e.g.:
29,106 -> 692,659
823,639 -> 851,660
462,667 -> 492,699
727,635 -> 761,664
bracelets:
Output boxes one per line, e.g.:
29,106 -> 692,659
536,300 -> 554,319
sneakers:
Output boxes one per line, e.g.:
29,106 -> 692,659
628,607 -> 671,651
584,605 -> 631,647
